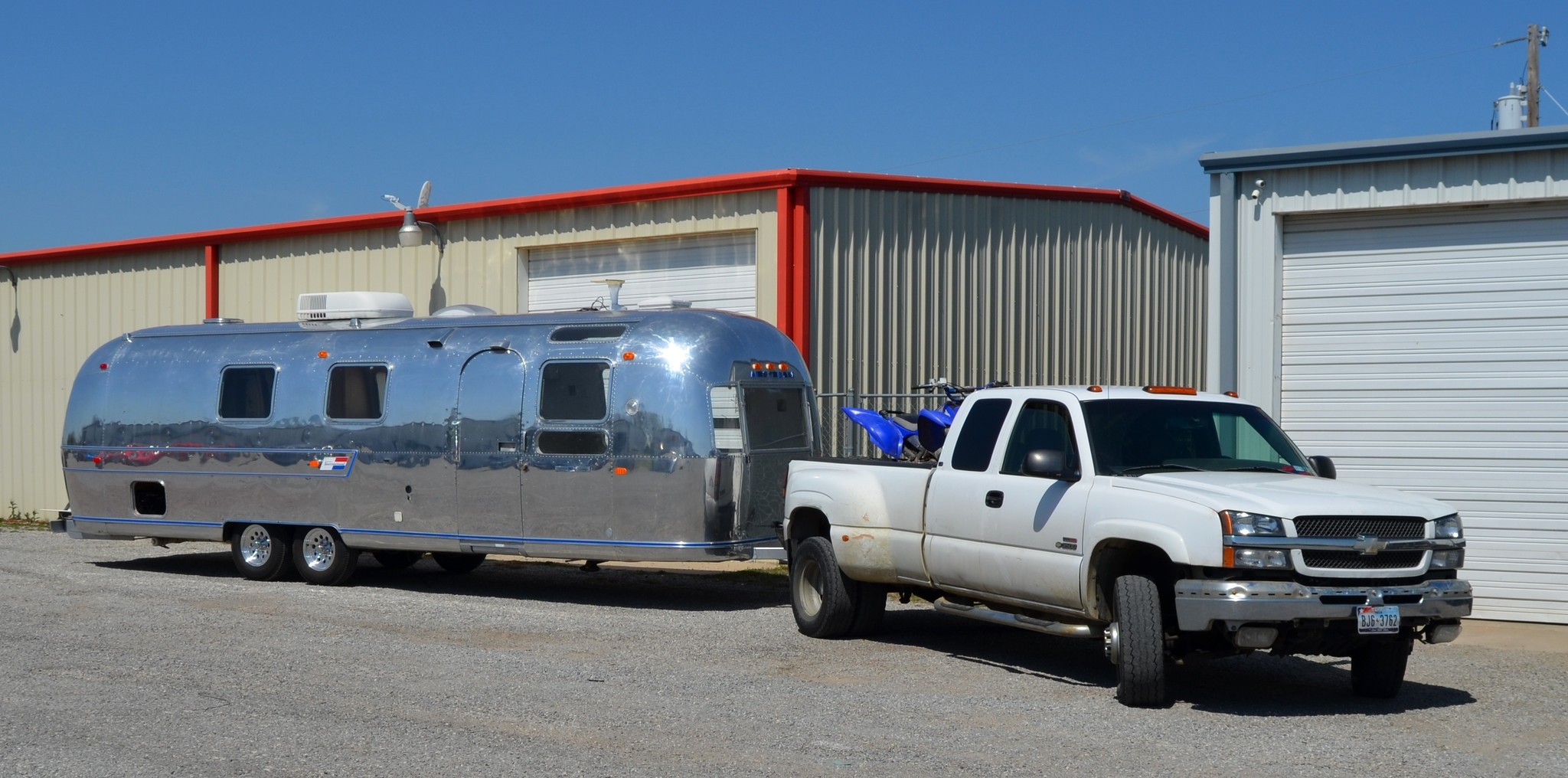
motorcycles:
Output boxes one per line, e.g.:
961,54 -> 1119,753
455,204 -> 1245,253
840,380 -> 1014,464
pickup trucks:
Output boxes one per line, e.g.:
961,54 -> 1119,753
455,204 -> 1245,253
777,384 -> 1472,709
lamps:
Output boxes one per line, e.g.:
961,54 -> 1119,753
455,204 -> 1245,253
399,209 -> 446,255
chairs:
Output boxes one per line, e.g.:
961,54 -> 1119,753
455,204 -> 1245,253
1027,427 -> 1064,451
1117,422 -> 1177,466
